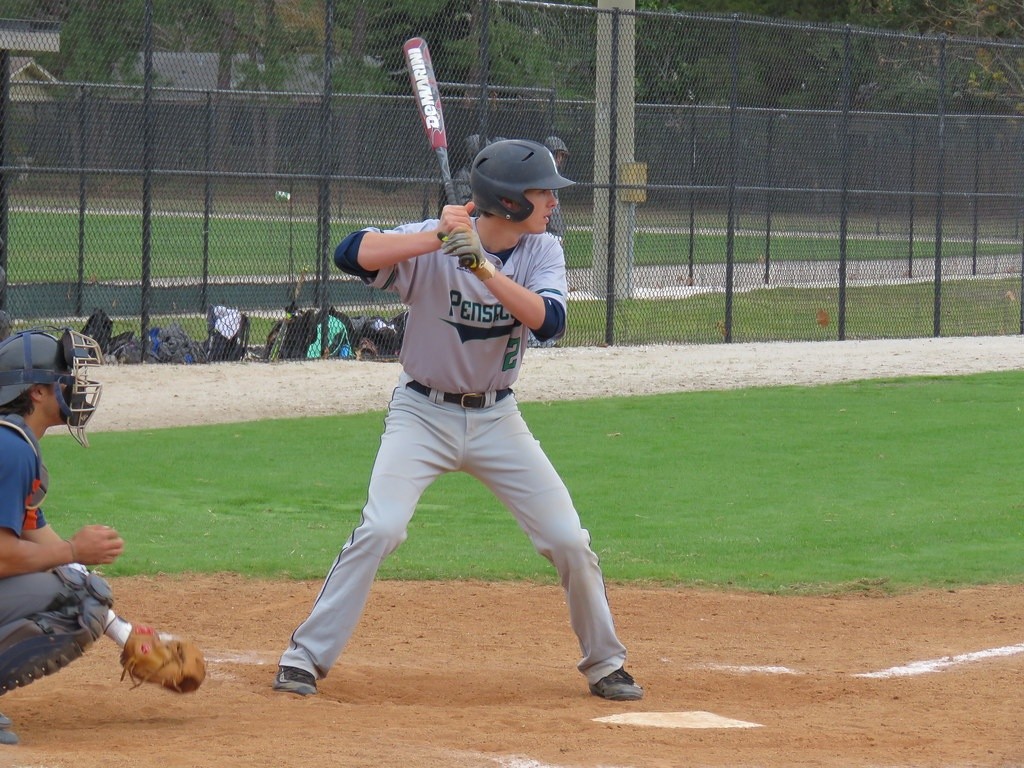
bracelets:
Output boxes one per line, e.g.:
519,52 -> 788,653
66,538 -> 76,563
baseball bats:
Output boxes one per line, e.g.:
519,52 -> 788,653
402,34 -> 474,268
268,267 -> 308,361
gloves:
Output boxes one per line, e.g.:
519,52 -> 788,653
441,227 -> 496,282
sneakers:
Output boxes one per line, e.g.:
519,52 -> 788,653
589,665 -> 642,700
272,662 -> 317,697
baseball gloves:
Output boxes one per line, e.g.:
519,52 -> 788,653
118,621 -> 205,696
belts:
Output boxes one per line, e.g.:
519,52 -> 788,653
407,378 -> 511,408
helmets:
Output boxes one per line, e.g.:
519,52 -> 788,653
0,328 -> 72,405
543,136 -> 569,153
470,140 -> 575,222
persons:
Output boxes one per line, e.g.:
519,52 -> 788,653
276,135 -> 644,702
0,326 -> 206,744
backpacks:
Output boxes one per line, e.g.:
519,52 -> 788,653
307,305 -> 357,360
80,309 -> 112,358
207,308 -> 250,362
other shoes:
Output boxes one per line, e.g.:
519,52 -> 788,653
0,712 -> 18,743
